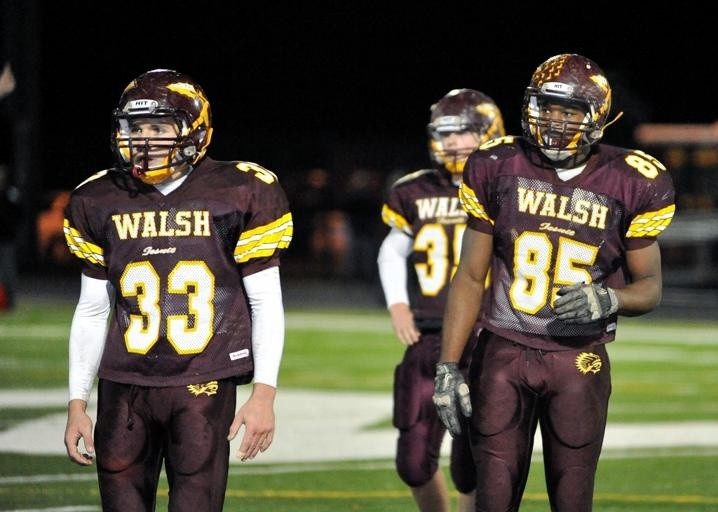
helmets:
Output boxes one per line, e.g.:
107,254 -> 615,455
110,69 -> 213,185
429,89 -> 504,173
522,53 -> 611,161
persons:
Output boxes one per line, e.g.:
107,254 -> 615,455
375,87 -> 505,510
431,53 -> 679,511
63,67 -> 296,511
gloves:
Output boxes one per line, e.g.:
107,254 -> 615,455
432,362 -> 472,437
554,283 -> 620,324
